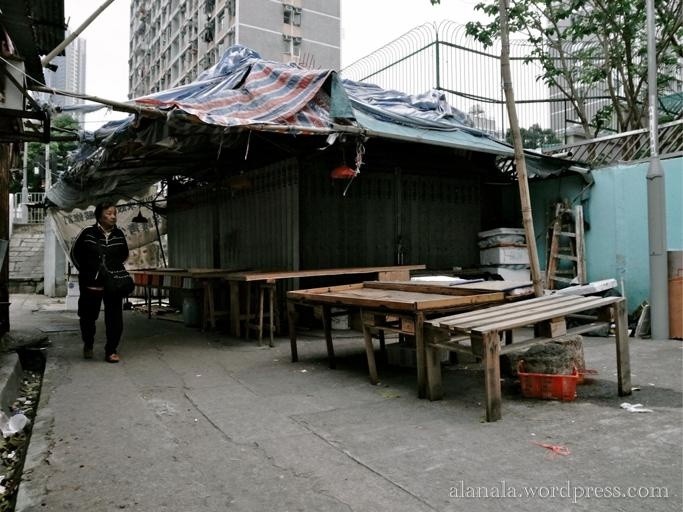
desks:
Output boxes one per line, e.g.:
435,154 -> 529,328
125,267 -> 426,348
423,292 -> 632,422
288,283 -> 504,400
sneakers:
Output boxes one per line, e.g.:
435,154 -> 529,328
105,353 -> 120,362
84,342 -> 93,359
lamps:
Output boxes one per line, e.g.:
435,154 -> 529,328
132,205 -> 148,222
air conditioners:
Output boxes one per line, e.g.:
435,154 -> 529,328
284,35 -> 291,40
295,38 -> 301,44
295,8 -> 302,14
284,6 -> 292,11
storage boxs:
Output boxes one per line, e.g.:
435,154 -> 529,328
516,360 -> 579,401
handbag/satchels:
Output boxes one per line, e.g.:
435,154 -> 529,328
95,260 -> 135,296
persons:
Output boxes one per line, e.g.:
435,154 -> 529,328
71,201 -> 129,362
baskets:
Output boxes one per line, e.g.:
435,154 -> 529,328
517,360 -> 585,401
133,273 -> 204,289
313,305 -> 323,319
385,342 -> 450,368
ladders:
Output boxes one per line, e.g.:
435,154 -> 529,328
546,203 -> 587,290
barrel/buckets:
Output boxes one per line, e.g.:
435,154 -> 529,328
668,276 -> 683,340
